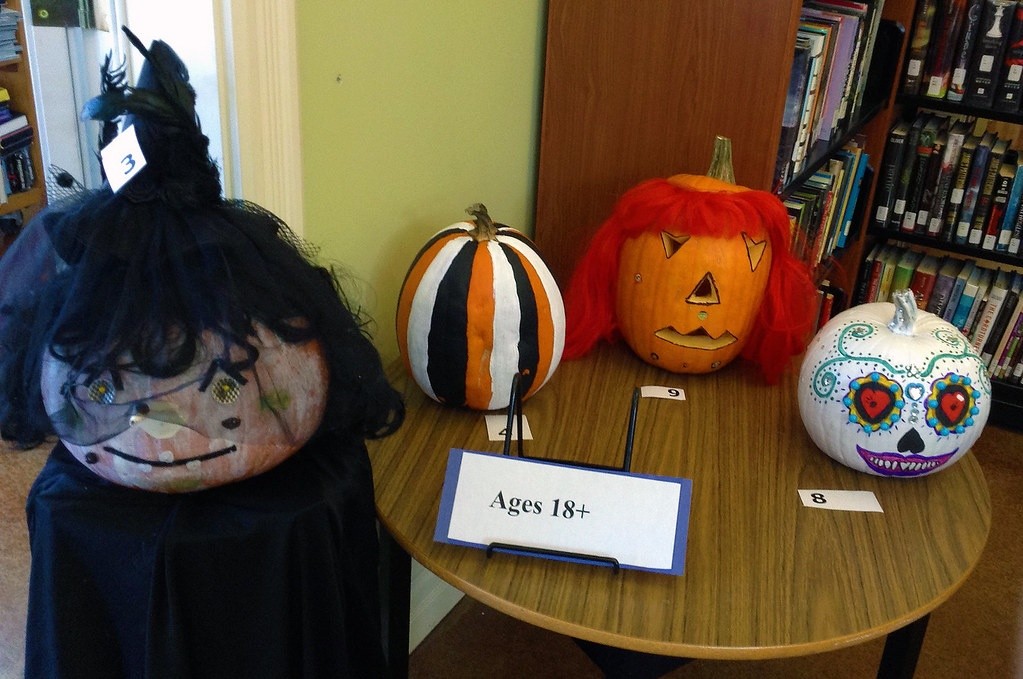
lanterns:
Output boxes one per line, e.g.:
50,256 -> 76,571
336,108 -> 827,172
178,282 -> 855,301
615,136 -> 771,375
41,315 -> 330,494
796,287 -> 992,477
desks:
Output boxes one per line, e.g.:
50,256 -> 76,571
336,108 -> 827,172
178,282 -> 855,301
26,445 -> 411,679
364,338 -> 994,679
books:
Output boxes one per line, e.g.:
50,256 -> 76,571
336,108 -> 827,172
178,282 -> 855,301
0,9 -> 35,204
774,0 -> 1023,386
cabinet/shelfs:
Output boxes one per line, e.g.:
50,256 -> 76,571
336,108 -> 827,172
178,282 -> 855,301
0,1 -> 51,259
535,1 -> 1023,430
